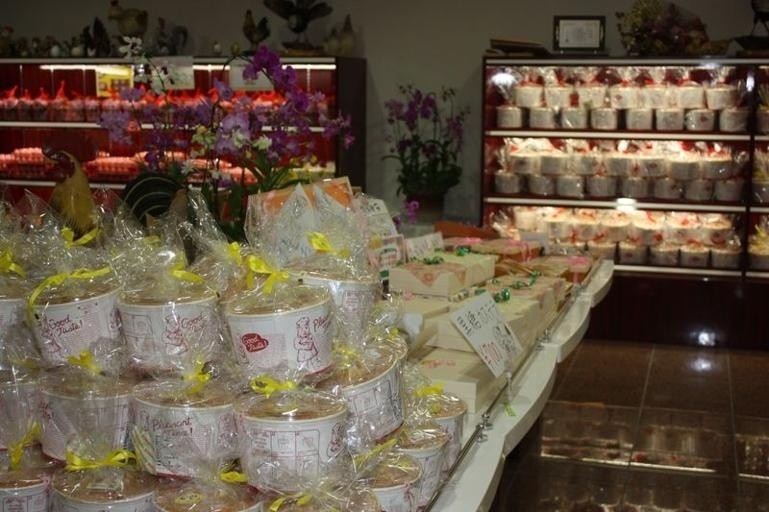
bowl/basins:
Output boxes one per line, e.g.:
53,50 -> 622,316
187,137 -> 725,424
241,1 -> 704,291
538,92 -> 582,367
495,105 -> 527,129
363,454 -> 425,512
511,84 -> 544,108
624,108 -> 653,130
528,107 -> 560,130
426,397 -> 468,473
390,422 -> 452,507
223,289 -> 335,386
655,109 -> 685,131
293,262 -> 382,346
718,108 -> 749,132
491,147 -> 769,204
559,107 -> 589,129
575,87 -> 606,109
336,344 -> 404,444
0,468 -> 51,512
118,287 -> 220,374
391,335 -> 412,373
704,86 -> 739,111
38,378 -> 134,464
642,86 -> 673,109
49,478 -> 162,512
498,208 -> 769,273
151,496 -> 267,512
27,285 -> 124,373
686,110 -> 715,133
259,499 -> 292,512
543,86 -> 574,108
756,108 -> 769,133
673,86 -> 705,109
0,377 -> 40,451
132,380 -> 238,478
609,84 -> 641,109
233,391 -> 348,498
0,294 -> 26,360
590,108 -> 619,130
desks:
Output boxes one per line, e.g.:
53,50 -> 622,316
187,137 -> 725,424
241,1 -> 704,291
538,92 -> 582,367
428,254 -> 614,512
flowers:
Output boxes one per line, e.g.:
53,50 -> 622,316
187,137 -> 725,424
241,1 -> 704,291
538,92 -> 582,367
386,81 -> 470,224
616,0 -> 709,54
97,38 -> 355,241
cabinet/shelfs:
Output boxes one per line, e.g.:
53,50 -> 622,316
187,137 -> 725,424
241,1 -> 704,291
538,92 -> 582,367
479,58 -> 768,352
0,54 -> 366,232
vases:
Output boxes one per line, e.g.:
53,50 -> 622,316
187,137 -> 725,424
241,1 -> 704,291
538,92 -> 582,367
404,196 -> 446,224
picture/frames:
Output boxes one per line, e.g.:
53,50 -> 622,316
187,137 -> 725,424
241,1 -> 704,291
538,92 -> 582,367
552,14 -> 606,51
